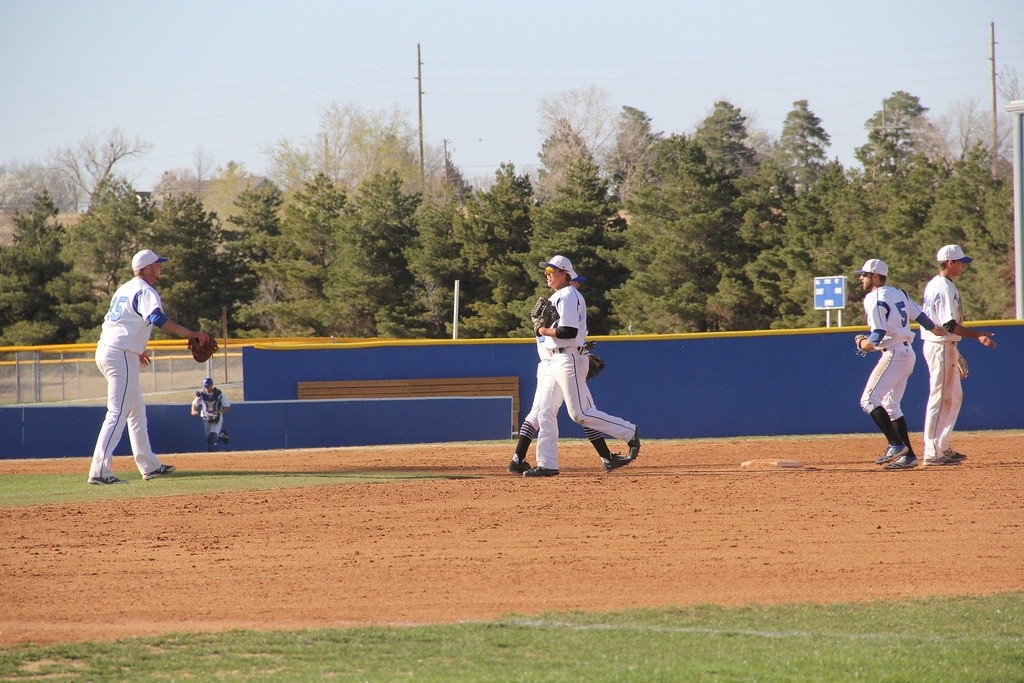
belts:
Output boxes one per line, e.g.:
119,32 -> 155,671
883,341 -> 908,352
553,347 -> 580,353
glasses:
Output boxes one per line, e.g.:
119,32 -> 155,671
858,272 -> 867,276
546,266 -> 563,273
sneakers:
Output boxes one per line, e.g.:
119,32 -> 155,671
508,460 -> 531,473
143,465 -> 175,480
884,456 -> 919,469
88,476 -> 129,485
924,454 -> 960,466
876,441 -> 909,466
628,425 -> 641,459
944,448 -> 967,460
603,452 -> 631,473
523,466 -> 560,477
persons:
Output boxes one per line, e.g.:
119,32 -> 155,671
854,259 -> 948,469
88,249 -> 209,485
506,255 -> 641,477
191,378 -> 231,451
920,245 -> 996,466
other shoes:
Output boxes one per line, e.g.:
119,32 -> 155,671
220,430 -> 231,439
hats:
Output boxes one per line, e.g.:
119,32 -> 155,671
568,269 -> 586,282
937,245 -> 973,263
854,259 -> 888,276
202,378 -> 212,386
539,255 -> 572,271
132,249 -> 168,271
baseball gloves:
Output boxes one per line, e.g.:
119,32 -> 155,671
530,297 -> 560,337
854,334 -> 871,352
956,354 -> 968,381
586,353 -> 604,382
188,333 -> 219,363
